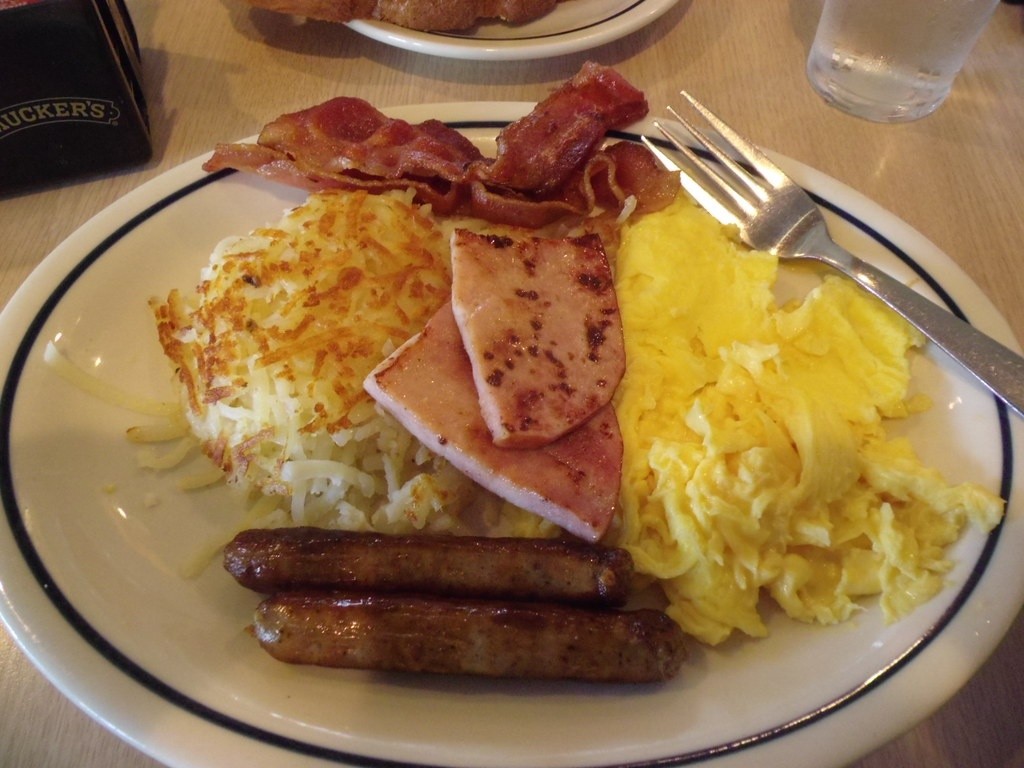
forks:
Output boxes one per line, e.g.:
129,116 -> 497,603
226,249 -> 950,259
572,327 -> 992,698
640,88 -> 1024,420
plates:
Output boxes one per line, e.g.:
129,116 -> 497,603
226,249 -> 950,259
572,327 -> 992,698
1,98 -> 1024,768
344,0 -> 680,61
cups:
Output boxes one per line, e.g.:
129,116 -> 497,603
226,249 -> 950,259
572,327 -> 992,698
805,0 -> 1001,123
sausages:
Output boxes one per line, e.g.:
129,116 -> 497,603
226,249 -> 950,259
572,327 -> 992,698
252,588 -> 687,685
224,525 -> 637,611
